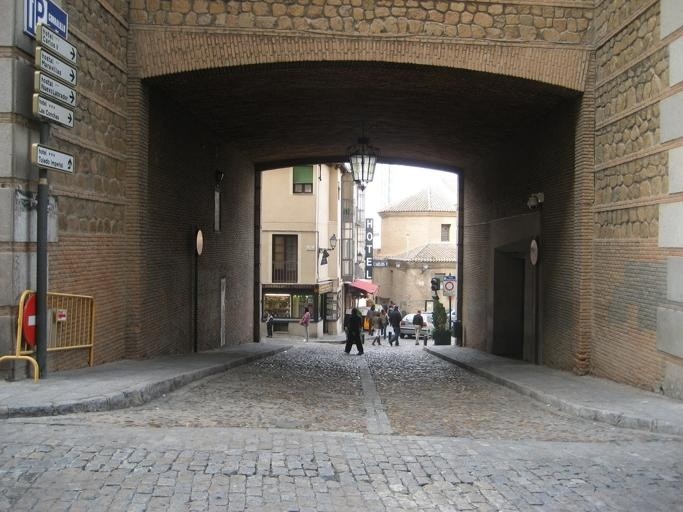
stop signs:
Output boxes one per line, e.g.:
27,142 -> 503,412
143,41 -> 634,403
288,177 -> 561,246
24,294 -> 37,347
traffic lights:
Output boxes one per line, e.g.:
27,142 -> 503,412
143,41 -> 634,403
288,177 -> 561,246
431,278 -> 440,290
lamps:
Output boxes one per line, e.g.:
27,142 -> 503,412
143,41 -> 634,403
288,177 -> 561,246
319,233 -> 338,254
347,108 -> 378,191
355,252 -> 364,265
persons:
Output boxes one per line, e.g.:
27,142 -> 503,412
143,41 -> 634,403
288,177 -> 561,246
343,308 -> 364,356
299,306 -> 310,343
411,310 -> 423,346
265,311 -> 274,338
365,301 -> 402,346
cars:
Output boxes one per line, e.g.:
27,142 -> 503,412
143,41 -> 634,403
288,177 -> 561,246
400,314 -> 432,338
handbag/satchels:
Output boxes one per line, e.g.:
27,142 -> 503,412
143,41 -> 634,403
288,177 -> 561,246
300,320 -> 303,325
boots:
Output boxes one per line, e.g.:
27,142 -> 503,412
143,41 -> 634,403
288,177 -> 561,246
372,335 -> 381,345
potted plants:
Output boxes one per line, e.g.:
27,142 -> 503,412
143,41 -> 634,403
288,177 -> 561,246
430,302 -> 451,345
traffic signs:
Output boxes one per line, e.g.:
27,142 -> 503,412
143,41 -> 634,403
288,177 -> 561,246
36,22 -> 78,69
35,46 -> 78,87
32,143 -> 75,175
34,71 -> 76,110
32,93 -> 76,129
